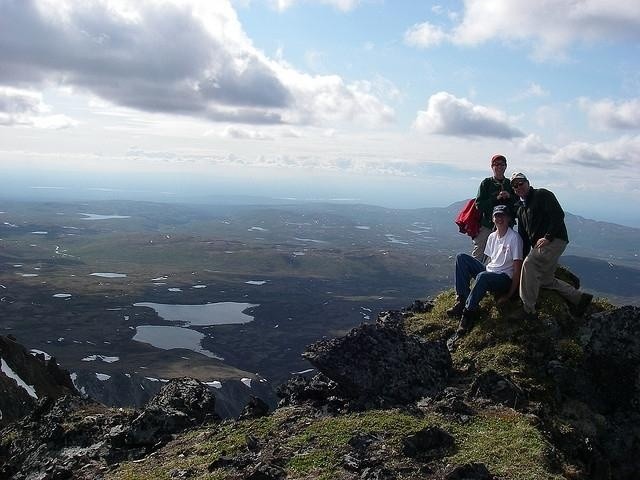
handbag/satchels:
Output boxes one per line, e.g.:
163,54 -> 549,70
456,198 -> 481,239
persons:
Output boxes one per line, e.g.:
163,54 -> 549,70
467,153 -> 522,266
507,170 -> 596,323
446,204 -> 525,336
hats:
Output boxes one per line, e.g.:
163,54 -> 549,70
492,155 -> 507,163
492,205 -> 509,216
510,172 -> 526,181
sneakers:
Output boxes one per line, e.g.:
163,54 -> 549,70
456,311 -> 474,338
508,308 -> 538,321
446,300 -> 465,317
574,292 -> 593,316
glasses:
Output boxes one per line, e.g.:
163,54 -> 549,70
512,179 -> 526,188
492,161 -> 506,167
